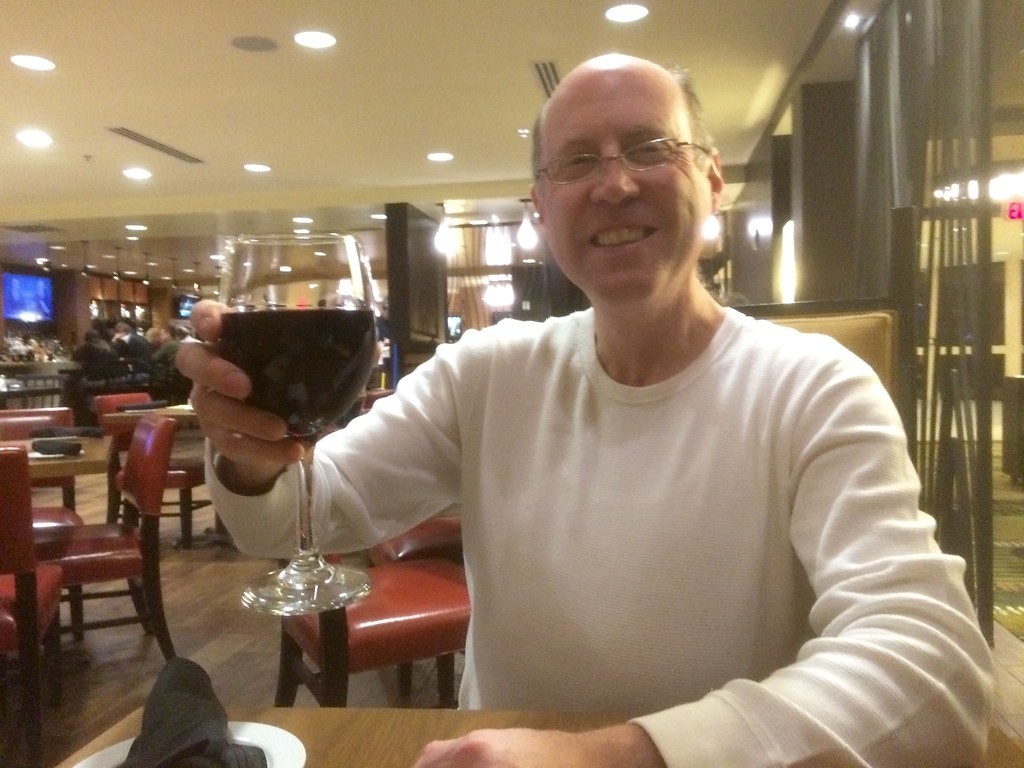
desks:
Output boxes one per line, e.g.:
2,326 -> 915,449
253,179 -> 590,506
100,405 -> 201,554
53,708 -> 1024,768
0,435 -> 115,648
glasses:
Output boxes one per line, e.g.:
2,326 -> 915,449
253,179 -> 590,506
533,137 -> 710,183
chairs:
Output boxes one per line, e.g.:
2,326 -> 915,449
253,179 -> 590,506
729,297 -> 917,470
0,390 -> 214,768
269,517 -> 472,710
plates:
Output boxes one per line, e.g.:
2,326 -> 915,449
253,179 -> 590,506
72,721 -> 306,768
28,450 -> 84,459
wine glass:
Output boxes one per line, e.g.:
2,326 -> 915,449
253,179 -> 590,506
217,232 -> 377,617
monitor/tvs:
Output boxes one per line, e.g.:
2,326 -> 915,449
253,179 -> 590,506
176,294 -> 200,318
0,262 -> 57,331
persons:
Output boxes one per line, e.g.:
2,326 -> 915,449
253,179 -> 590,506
65,312 -> 197,426
376,295 -> 394,371
173,51 -> 998,768
